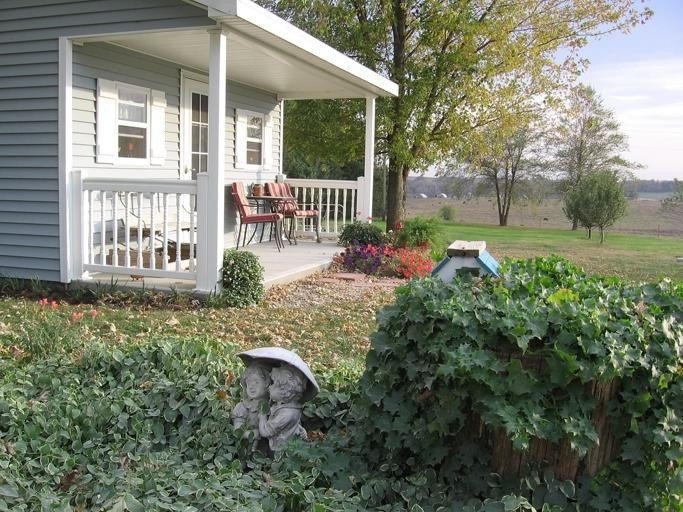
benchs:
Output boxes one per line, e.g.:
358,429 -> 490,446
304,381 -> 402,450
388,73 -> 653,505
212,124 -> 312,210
109,190 -> 198,263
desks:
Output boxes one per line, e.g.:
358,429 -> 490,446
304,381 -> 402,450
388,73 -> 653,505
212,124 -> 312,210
246,193 -> 295,250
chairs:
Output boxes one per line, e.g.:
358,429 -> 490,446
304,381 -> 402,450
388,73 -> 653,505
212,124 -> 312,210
263,182 -> 323,244
228,181 -> 285,252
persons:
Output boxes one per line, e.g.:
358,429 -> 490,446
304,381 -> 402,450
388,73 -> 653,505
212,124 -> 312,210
258,365 -> 309,458
230,359 -> 271,452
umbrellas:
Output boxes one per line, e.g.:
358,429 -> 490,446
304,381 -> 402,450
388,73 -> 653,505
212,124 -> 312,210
236,346 -> 320,405
